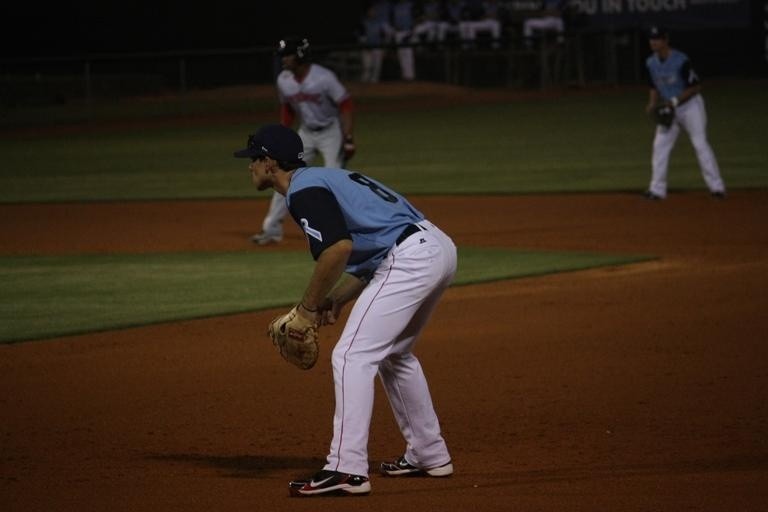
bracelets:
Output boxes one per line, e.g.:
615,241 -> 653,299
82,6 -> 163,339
300,301 -> 318,312
671,96 -> 679,107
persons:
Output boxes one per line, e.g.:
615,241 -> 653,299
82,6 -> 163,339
356,0 -> 568,83
642,22 -> 727,199
250,28 -> 356,246
233,120 -> 459,496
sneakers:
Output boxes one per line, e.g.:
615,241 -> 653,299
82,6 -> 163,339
711,192 -> 727,202
287,473 -> 372,497
639,190 -> 659,200
379,452 -> 455,477
250,232 -> 283,246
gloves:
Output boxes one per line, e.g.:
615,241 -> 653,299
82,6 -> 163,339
339,134 -> 355,162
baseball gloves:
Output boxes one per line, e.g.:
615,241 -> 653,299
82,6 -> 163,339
649,100 -> 676,130
267,301 -> 321,370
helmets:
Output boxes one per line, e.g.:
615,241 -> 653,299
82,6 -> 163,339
269,33 -> 309,69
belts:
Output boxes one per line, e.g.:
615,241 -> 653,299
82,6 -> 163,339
395,223 -> 426,247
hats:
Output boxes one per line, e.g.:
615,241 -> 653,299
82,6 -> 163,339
233,123 -> 303,161
649,24 -> 666,38
